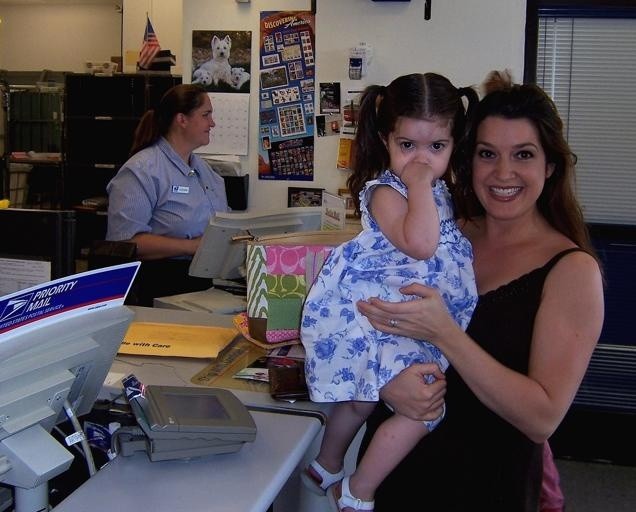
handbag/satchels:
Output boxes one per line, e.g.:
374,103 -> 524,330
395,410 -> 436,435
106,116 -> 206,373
243,241 -> 338,345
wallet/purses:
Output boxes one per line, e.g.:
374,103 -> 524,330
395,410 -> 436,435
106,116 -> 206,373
268,365 -> 309,402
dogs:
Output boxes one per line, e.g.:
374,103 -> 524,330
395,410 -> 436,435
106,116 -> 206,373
191,68 -> 213,87
199,34 -> 234,86
230,67 -> 250,91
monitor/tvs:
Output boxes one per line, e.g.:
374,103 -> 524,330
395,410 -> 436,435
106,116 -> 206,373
0,304 -> 136,512
186,206 -> 324,288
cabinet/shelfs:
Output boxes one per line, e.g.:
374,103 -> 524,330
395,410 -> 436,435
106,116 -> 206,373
0,69 -> 183,216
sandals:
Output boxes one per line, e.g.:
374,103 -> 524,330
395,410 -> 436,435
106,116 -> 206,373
300,459 -> 374,511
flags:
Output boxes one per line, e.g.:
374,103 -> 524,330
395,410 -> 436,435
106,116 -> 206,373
136,16 -> 160,70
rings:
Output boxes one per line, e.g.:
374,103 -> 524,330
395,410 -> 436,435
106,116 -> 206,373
389,319 -> 400,327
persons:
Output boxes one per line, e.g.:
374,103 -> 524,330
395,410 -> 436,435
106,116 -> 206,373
106,85 -> 232,308
356,83 -> 606,511
299,73 -> 479,510
262,136 -> 270,150
